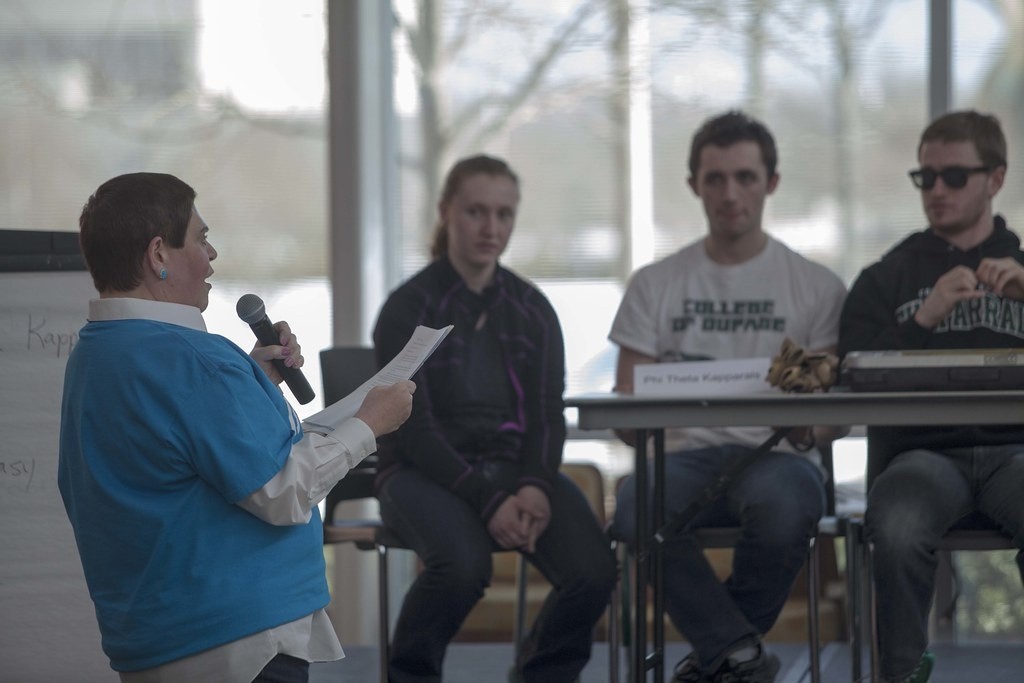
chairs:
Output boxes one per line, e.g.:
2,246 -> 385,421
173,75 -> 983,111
317,346 -> 529,683
868,523 -> 1022,682
605,511 -> 864,680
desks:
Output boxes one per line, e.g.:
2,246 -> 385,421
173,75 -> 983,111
558,387 -> 1023,682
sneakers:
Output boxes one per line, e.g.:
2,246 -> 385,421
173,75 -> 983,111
668,642 -> 782,682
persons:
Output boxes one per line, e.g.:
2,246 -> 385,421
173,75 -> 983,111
608,106 -> 849,682
374,154 -> 616,682
837,109 -> 1024,683
58,173 -> 417,683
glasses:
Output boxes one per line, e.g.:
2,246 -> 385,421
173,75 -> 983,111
908,164 -> 991,191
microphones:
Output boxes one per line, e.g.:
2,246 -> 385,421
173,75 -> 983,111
235,293 -> 316,405
